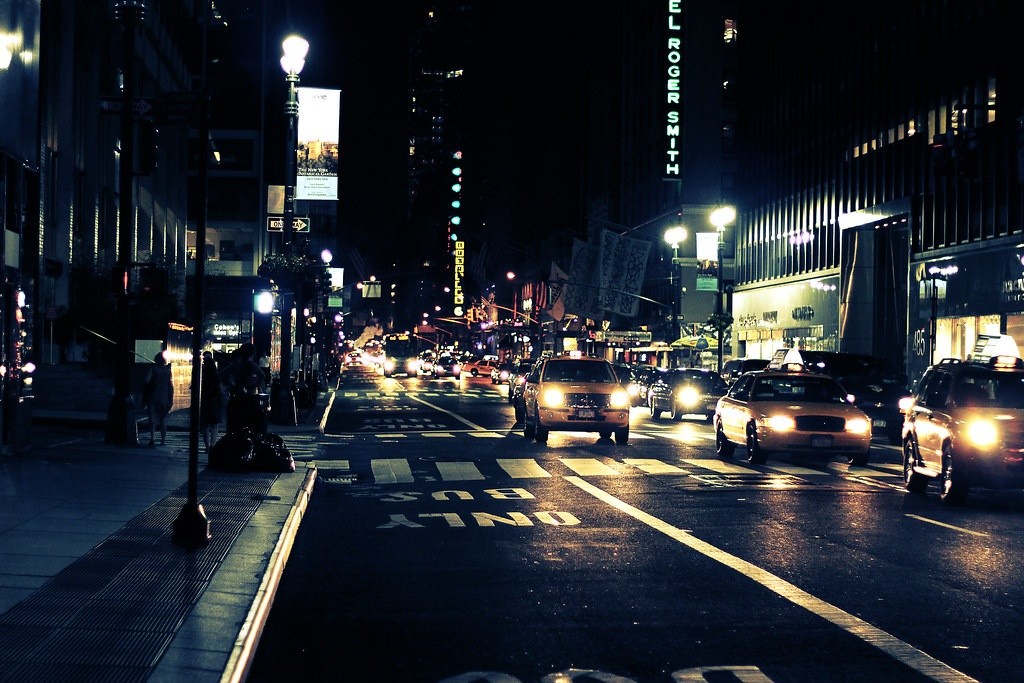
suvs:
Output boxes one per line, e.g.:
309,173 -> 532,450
901,357 -> 1024,512
720,358 -> 771,389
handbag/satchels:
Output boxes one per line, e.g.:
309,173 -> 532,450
141,366 -> 156,404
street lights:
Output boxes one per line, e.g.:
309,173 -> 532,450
268,32 -> 311,428
706,204 -> 738,376
663,225 -> 689,370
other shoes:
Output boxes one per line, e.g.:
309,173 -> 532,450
148,440 -> 154,446
161,443 -> 166,447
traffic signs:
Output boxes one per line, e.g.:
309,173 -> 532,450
665,314 -> 685,321
266,216 -> 311,233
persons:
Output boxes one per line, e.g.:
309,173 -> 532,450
199,354 -> 222,452
140,353 -> 173,447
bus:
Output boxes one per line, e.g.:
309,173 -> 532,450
381,332 -> 417,377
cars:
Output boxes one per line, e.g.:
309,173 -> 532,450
712,347 -> 873,468
647,368 -> 734,423
611,361 -> 668,408
421,347 -> 536,425
523,350 -> 631,444
799,349 -> 912,446
